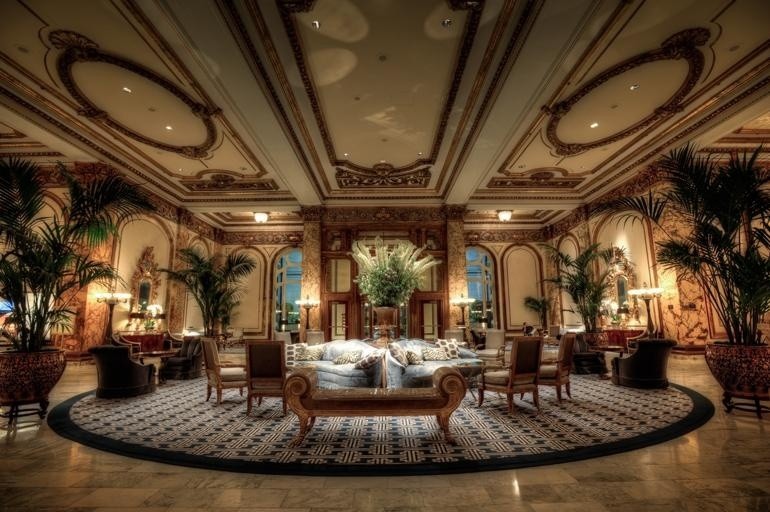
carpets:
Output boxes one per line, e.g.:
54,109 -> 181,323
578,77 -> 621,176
48,360 -> 717,479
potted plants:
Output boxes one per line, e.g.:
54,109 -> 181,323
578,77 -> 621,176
580,137 -> 769,406
0,147 -> 158,410
537,243 -> 632,350
347,233 -> 441,346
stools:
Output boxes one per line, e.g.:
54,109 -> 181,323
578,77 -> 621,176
283,367 -> 466,446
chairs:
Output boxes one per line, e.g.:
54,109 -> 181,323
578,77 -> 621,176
477,322 -> 589,414
297,338 -> 483,393
243,339 -> 290,417
88,327 -> 256,400
568,327 -> 607,374
609,336 -> 678,392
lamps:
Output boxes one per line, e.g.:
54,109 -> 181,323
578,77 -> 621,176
251,211 -> 270,226
93,291 -> 130,343
625,280 -> 664,334
295,295 -> 320,329
496,208 -> 515,224
451,290 -> 476,329
